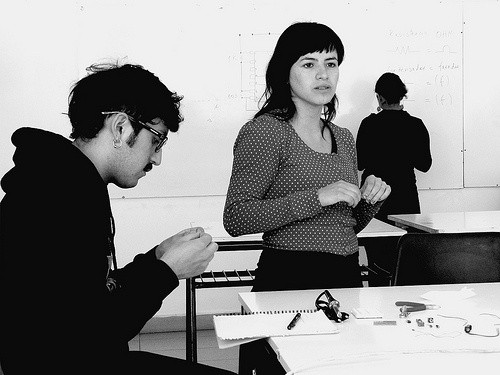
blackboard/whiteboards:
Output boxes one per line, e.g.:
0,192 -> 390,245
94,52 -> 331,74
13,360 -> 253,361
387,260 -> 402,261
0,0 -> 500,199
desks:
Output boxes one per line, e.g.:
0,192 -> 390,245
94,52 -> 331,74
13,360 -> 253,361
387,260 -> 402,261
387,211 -> 500,233
186,218 -> 407,362
237,282 -> 500,375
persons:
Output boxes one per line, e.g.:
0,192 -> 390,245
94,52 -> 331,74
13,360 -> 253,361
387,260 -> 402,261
223,22 -> 392,375
0,62 -> 243,375
356,72 -> 433,288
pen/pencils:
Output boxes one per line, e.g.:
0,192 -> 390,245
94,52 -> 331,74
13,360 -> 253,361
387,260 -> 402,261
287,313 -> 301,330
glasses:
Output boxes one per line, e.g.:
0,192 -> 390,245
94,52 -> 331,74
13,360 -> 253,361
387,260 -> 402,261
102,111 -> 169,153
315,290 -> 350,324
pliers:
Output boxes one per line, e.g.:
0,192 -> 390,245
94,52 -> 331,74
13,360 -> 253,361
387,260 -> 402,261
396,301 -> 440,312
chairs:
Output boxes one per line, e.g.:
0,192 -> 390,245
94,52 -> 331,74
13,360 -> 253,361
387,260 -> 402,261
391,232 -> 500,286
